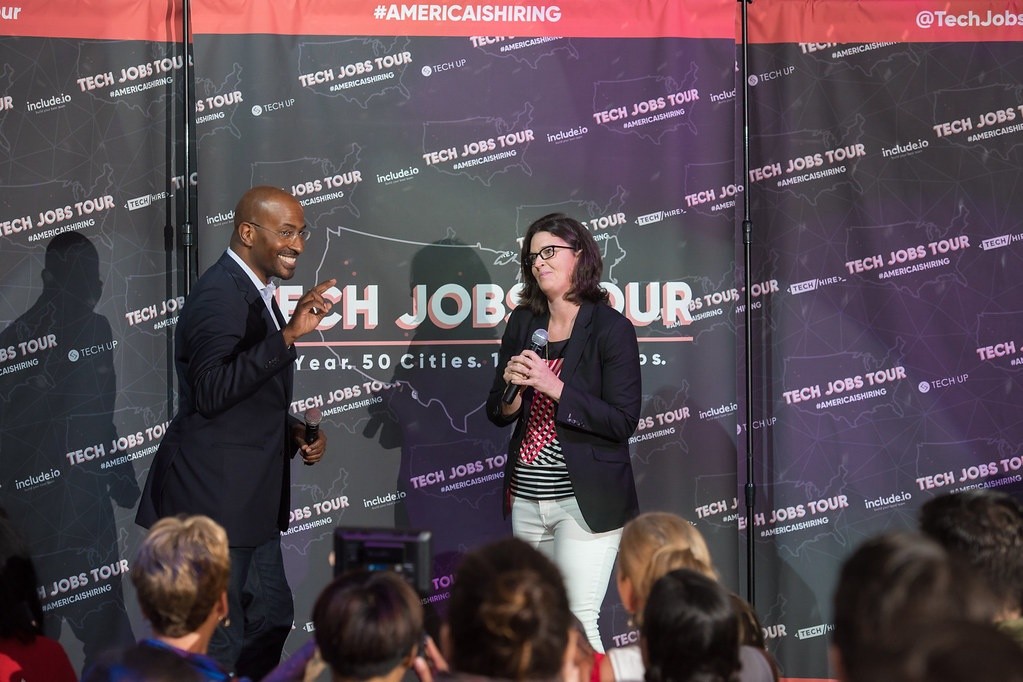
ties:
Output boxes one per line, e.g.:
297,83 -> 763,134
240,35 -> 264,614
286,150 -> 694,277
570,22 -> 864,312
263,288 -> 282,331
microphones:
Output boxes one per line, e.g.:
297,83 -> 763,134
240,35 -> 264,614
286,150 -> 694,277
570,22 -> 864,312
502,329 -> 549,405
304,408 -> 322,465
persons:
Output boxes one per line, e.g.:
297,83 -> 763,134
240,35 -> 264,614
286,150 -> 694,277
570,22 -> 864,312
79,514 -> 246,681
135,187 -> 337,674
485,215 -> 644,654
0,526 -> 80,682
302,537 -> 617,682
615,512 -> 780,682
826,489 -> 1023,682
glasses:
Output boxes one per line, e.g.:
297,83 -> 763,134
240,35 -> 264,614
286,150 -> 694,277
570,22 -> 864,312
251,222 -> 312,243
524,245 -> 575,265
